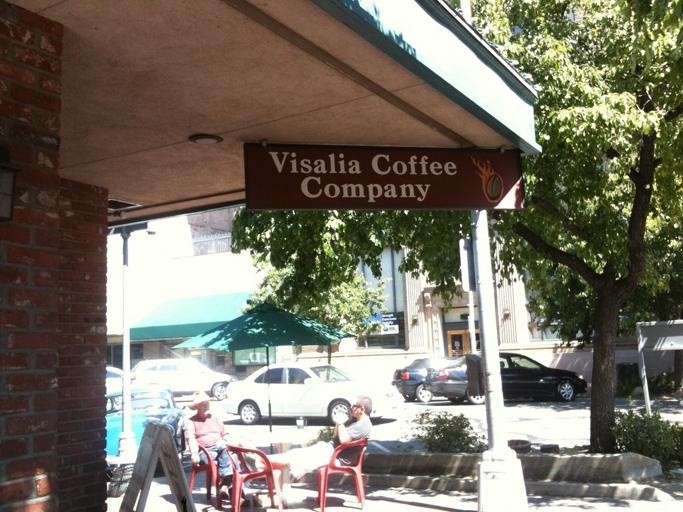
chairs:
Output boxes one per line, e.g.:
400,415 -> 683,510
186,427 -> 222,504
225,445 -> 288,511
316,437 -> 367,512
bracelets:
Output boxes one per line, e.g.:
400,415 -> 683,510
337,423 -> 344,427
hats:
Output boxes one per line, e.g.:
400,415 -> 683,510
187,390 -> 216,410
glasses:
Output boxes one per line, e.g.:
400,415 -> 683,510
351,403 -> 362,411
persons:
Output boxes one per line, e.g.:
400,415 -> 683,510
184,391 -> 263,503
285,398 -> 372,482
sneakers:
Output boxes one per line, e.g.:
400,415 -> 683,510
219,485 -> 229,500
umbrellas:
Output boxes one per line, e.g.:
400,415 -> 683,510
171,300 -> 358,432
